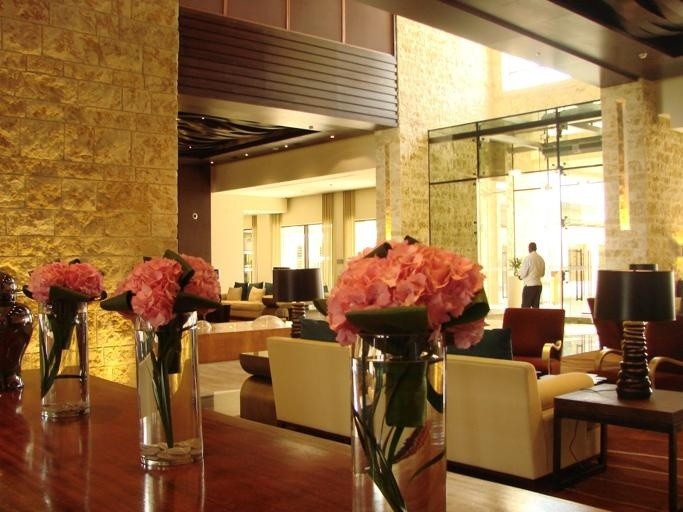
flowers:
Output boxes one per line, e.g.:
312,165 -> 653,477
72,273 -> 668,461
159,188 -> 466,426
98,247 -> 229,448
20,257 -> 108,399
323,232 -> 491,512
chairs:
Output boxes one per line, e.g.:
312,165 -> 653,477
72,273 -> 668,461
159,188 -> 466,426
586,298 -> 622,355
500,304 -> 566,379
577,317 -> 682,390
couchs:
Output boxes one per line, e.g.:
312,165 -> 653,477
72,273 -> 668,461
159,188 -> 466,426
217,284 -> 278,319
262,331 -> 607,485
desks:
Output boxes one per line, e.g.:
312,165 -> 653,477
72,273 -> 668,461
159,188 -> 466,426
0,367 -> 609,512
550,381 -> 682,512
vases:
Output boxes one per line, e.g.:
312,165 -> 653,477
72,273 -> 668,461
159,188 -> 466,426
0,271 -> 34,393
346,333 -> 451,512
33,301 -> 90,421
131,318 -> 207,470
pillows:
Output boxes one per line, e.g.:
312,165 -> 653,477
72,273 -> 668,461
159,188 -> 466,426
444,323 -> 513,360
264,281 -> 272,296
246,285 -> 266,304
225,287 -> 243,301
247,282 -> 264,300
233,280 -> 248,301
297,316 -> 338,343
311,297 -> 326,315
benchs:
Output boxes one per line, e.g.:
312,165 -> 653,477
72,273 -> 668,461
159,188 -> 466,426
236,349 -> 290,426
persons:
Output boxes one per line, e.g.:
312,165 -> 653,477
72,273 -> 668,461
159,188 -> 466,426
518,242 -> 545,309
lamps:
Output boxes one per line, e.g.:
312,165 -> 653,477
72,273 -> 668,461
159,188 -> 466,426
271,266 -> 325,337
592,267 -> 678,401
